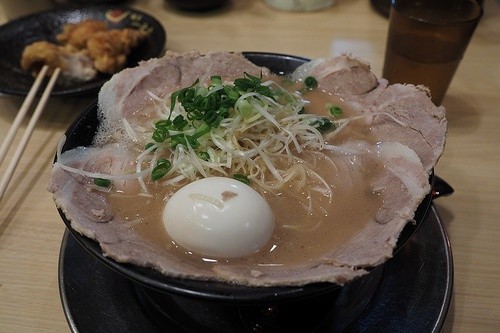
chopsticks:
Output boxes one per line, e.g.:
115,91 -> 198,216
0,62 -> 58,200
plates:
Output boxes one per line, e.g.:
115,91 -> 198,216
0,6 -> 167,99
58,204 -> 463,333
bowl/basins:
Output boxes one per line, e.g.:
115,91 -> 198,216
49,51 -> 454,332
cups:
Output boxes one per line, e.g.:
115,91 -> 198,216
382,1 -> 485,107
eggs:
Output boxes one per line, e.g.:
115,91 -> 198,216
162,177 -> 275,257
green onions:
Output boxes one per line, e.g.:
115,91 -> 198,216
92,70 -> 344,185
